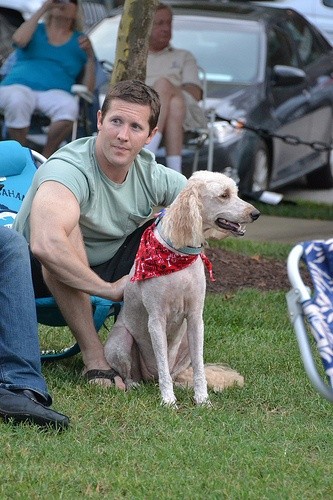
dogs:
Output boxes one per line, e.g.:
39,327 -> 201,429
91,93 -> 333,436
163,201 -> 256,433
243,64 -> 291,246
103,168 -> 262,409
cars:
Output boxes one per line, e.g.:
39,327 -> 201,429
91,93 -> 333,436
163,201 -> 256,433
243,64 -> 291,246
29,2 -> 333,201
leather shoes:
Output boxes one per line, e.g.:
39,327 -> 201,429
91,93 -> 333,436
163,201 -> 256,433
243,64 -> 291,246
0,385 -> 69,431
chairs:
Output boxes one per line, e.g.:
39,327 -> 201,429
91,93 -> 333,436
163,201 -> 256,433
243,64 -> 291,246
159,66 -> 215,175
0,50 -> 112,144
284,239 -> 333,403
0,140 -> 123,363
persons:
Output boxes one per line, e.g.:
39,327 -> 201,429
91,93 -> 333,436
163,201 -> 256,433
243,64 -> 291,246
0,0 -> 97,160
143,4 -> 205,174
13,81 -> 187,392
0,224 -> 70,436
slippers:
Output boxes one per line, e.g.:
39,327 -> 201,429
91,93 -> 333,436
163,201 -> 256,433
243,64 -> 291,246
81,366 -> 127,393
